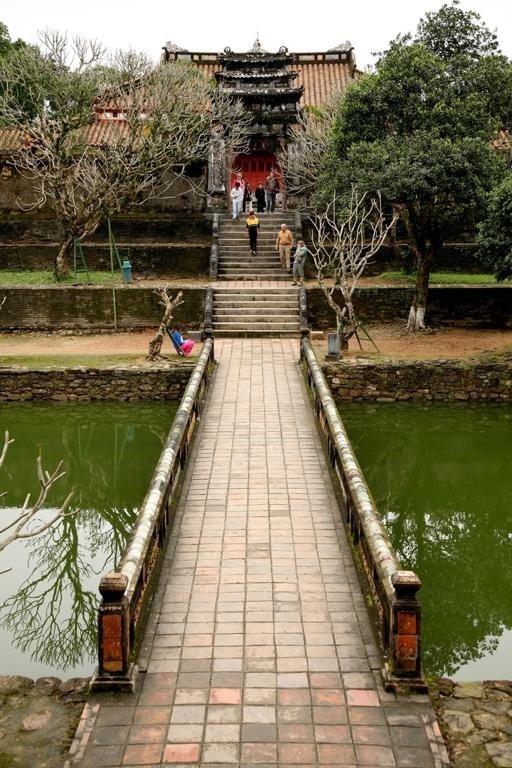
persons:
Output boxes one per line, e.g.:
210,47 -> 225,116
233,173 -> 245,213
170,324 -> 195,357
230,182 -> 243,224
246,211 -> 261,255
292,239 -> 308,286
244,182 -> 253,212
255,182 -> 266,213
265,172 -> 279,213
276,223 -> 293,272
